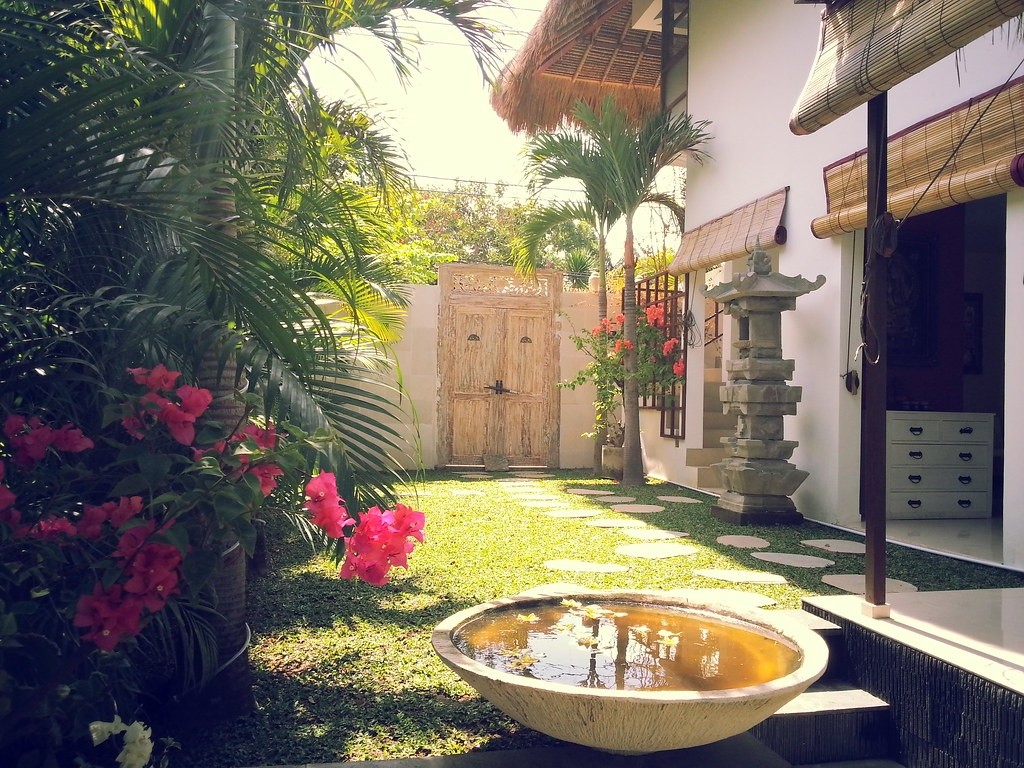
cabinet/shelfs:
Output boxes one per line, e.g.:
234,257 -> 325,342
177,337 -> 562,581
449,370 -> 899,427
886,410 -> 995,520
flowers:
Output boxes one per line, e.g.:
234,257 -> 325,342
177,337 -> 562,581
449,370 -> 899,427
591,304 -> 686,409
0,363 -> 424,768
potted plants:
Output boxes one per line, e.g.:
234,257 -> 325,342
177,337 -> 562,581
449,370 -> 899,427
581,384 -> 642,479
